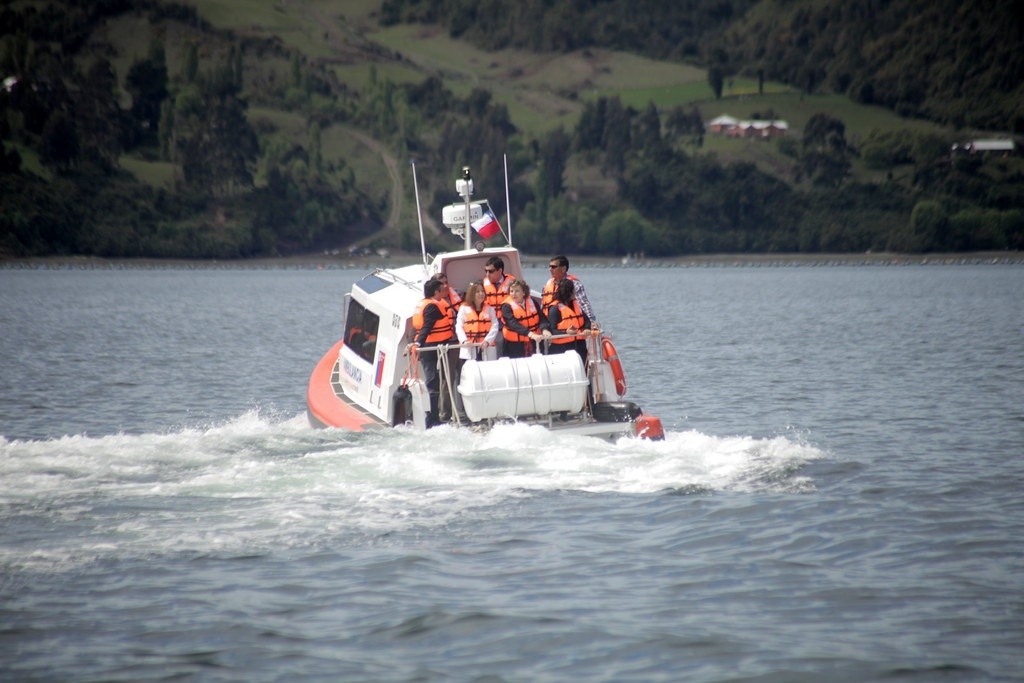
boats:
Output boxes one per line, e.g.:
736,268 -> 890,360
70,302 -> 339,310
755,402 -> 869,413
304,152 -> 665,444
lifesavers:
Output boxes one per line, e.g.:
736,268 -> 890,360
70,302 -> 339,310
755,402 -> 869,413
600,336 -> 626,395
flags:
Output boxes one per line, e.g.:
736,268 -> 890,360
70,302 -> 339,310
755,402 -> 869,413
471,211 -> 500,239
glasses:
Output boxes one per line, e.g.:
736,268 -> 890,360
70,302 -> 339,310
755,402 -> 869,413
549,265 -> 564,268
485,268 -> 496,273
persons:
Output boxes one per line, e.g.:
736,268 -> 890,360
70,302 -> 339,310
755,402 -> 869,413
403,256 -> 600,421
